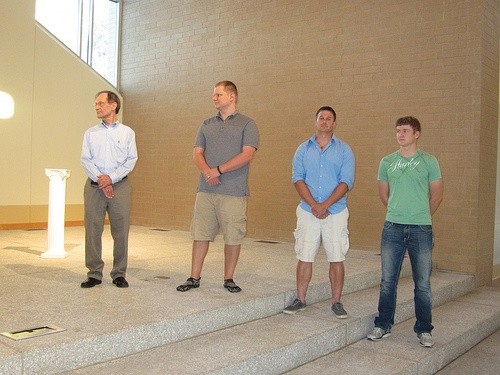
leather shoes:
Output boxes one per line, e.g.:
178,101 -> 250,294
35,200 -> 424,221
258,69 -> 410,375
113,277 -> 129,287
81,277 -> 102,288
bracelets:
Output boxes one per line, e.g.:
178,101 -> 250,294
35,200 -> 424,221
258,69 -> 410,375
217,165 -> 222,175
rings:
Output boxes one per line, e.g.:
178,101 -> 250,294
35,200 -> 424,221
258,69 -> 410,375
208,175 -> 210,178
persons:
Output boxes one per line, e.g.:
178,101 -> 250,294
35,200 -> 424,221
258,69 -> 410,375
80,91 -> 138,288
177,80 -> 258,293
283,106 -> 356,318
368,116 -> 442,347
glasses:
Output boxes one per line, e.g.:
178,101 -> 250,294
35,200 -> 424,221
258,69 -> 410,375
96,102 -> 113,106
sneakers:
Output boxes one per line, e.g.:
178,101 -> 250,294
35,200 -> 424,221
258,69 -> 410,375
367,326 -> 392,341
283,299 -> 307,314
331,303 -> 348,319
418,332 -> 434,347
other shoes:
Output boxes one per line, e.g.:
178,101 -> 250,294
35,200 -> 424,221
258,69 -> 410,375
176,277 -> 202,292
223,279 -> 242,293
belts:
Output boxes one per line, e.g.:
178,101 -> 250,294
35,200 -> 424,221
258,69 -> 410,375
91,176 -> 128,186
